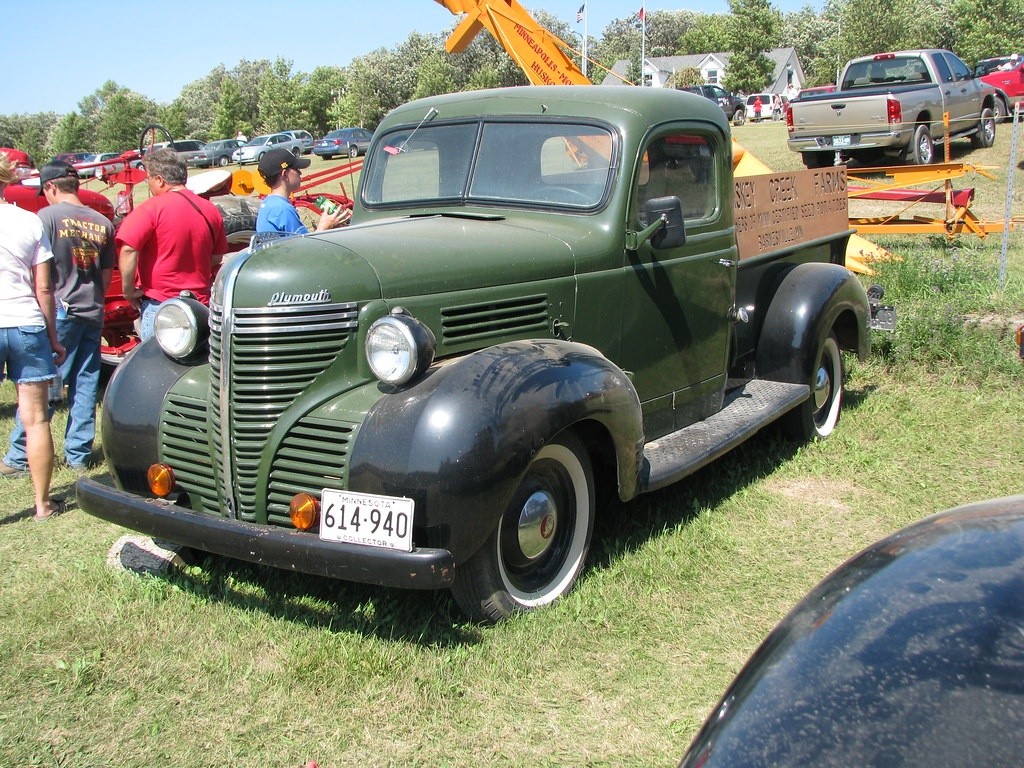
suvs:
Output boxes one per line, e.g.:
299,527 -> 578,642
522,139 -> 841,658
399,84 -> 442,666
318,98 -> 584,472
677,85 -> 746,126
280,130 -> 315,155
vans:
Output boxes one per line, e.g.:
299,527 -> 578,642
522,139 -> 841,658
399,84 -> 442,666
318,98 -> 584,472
745,93 -> 789,122
147,139 -> 206,162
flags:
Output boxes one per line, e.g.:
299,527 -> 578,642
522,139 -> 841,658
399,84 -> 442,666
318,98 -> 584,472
639,7 -> 646,20
576,4 -> 585,23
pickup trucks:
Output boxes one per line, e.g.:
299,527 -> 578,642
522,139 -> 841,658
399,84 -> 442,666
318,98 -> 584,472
785,48 -> 997,170
74,84 -> 897,630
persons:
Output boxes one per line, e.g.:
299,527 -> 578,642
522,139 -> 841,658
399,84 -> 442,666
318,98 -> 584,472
1001,54 -> 1019,71
774,94 -> 784,120
787,83 -> 798,102
0,147 -> 351,523
753,97 -> 762,122
237,131 -> 247,146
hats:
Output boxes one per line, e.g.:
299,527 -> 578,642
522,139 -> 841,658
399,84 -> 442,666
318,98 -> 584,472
756,97 -> 760,100
258,148 -> 311,179
1010,54 -> 1018,60
788,84 -> 794,87
37,160 -> 79,196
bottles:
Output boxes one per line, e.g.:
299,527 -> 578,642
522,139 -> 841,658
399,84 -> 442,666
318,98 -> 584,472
314,195 -> 350,225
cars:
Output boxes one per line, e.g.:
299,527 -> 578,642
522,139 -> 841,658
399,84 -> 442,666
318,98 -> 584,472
313,127 -> 373,160
796,85 -> 837,98
186,138 -> 248,169
973,58 -> 1024,125
56,147 -> 147,186
231,134 -> 305,166
0,124 -> 365,388
673,497 -> 1024,768
394,140 -> 437,154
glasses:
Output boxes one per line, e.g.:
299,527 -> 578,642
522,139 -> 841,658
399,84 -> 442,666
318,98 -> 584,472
144,174 -> 164,183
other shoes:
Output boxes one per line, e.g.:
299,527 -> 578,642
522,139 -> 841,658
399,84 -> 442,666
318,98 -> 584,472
0,461 -> 32,479
66,460 -> 85,470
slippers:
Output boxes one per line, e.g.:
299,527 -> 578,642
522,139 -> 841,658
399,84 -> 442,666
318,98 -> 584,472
34,500 -> 73,523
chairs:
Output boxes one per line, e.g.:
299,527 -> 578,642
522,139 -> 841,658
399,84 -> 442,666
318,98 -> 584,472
871,67 -> 886,81
920,66 -> 929,80
854,78 -> 869,85
905,73 -> 922,81
644,156 -> 709,218
